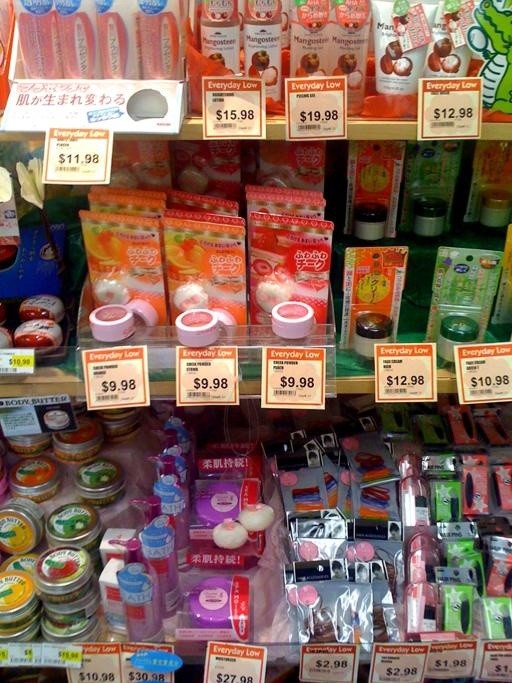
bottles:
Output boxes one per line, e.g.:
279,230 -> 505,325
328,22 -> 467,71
198,15 -> 283,100
353,195 -> 510,361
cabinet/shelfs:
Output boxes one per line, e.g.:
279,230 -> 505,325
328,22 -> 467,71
0,116 -> 512,679
